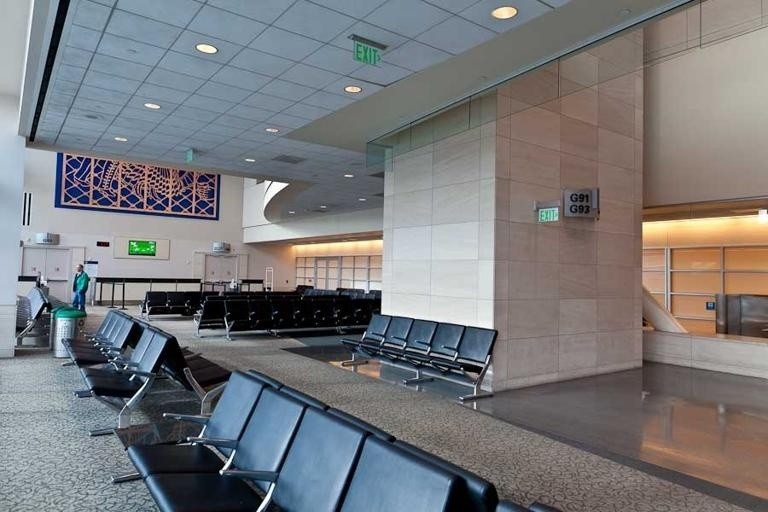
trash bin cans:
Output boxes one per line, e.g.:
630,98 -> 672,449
49,307 -> 87,358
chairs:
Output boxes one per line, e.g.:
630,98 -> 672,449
16,283 -> 92,351
337,313 -> 501,404
138,283 -> 381,344
112,365 -> 565,512
56,307 -> 235,438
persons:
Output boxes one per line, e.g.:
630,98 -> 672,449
72,263 -> 90,311
